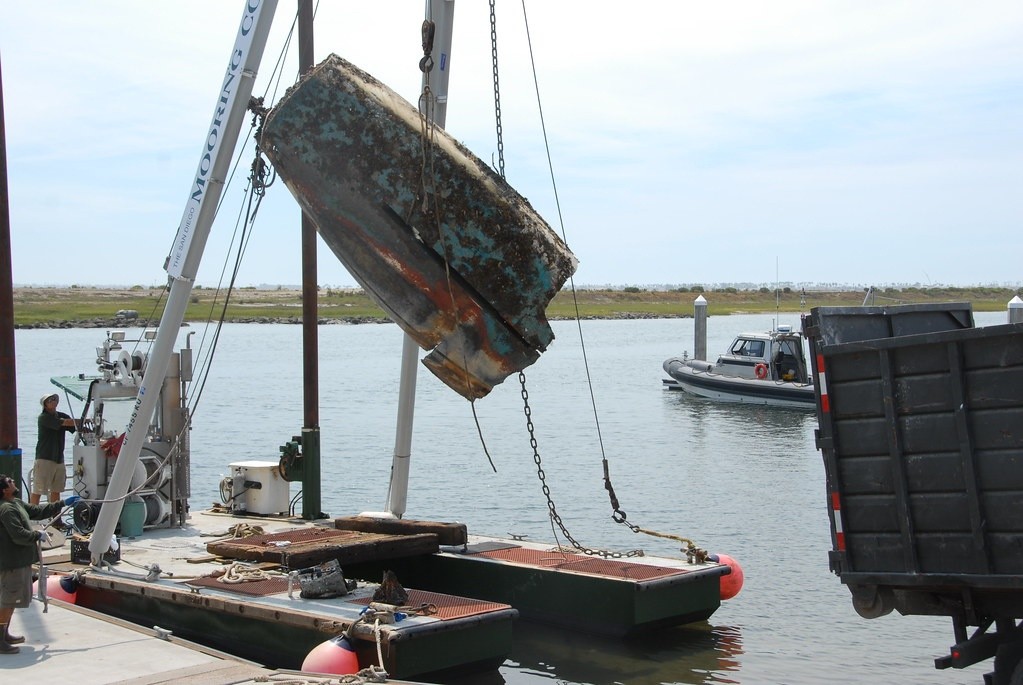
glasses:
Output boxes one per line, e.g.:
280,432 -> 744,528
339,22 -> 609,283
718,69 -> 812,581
2,478 -> 12,489
46,398 -> 56,404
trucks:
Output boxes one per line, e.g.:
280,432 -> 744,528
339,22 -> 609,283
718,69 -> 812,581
801,302 -> 1023,684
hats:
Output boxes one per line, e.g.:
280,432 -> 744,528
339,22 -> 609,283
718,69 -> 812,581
39,393 -> 59,414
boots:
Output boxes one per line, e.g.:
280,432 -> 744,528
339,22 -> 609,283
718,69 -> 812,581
5,621 -> 25,644
0,622 -> 20,654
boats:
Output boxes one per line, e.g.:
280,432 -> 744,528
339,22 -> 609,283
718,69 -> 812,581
662,294 -> 814,413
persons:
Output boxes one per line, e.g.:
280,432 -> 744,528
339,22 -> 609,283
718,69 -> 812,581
31,393 -> 94,527
0,474 -> 81,654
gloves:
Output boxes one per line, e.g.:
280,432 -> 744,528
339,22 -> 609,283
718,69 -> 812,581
37,529 -> 46,542
65,495 -> 80,507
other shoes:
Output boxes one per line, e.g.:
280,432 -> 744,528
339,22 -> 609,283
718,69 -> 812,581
53,517 -> 67,529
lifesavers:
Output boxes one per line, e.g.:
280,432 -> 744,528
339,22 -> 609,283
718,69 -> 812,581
755,364 -> 768,379
